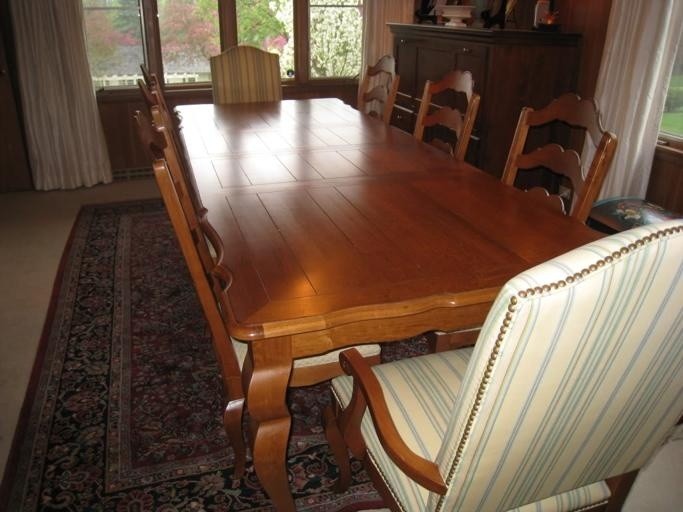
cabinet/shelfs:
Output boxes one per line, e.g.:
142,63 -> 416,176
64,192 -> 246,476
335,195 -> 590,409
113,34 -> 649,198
383,17 -> 582,195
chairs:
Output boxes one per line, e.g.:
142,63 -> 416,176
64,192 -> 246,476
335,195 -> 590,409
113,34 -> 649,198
317,220 -> 682,512
412,67 -> 481,162
137,80 -> 221,284
136,110 -> 380,486
138,63 -> 181,154
587,149 -> 683,237
360,56 -> 400,124
504,93 -> 619,228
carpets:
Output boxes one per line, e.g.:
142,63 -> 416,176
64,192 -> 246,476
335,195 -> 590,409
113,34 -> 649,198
0,196 -> 432,512
